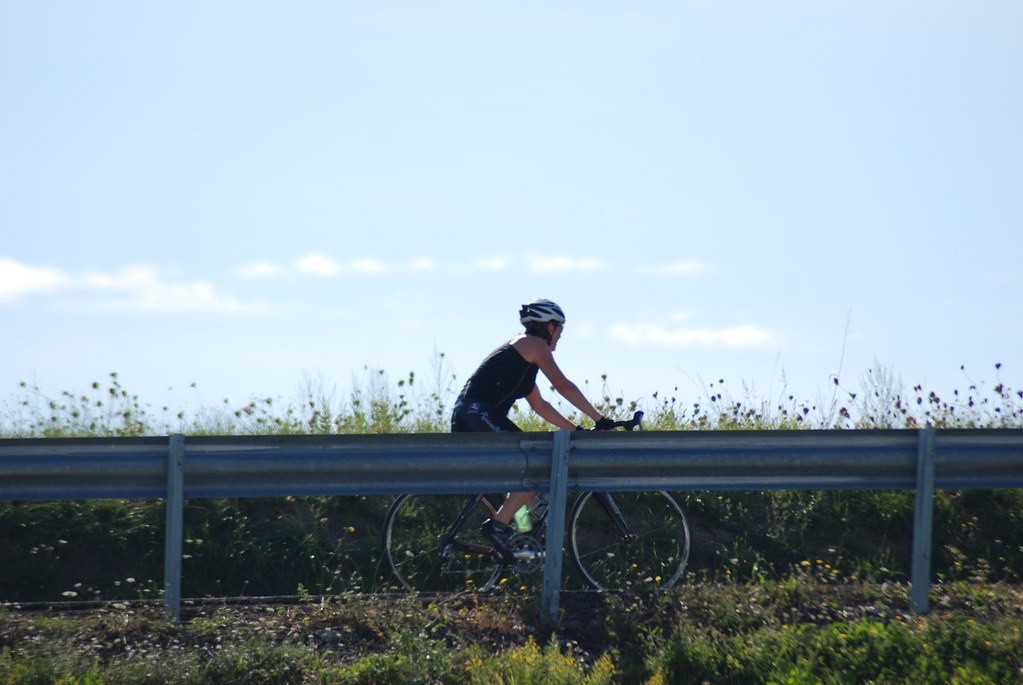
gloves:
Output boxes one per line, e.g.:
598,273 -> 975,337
594,415 -> 615,430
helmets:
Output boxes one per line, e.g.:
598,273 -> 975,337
518,298 -> 565,325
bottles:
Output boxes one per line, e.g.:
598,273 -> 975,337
506,492 -> 532,533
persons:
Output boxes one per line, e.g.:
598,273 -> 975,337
450,300 -> 614,565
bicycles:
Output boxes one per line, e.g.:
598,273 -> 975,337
381,412 -> 694,593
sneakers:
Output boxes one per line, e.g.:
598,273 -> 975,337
479,518 -> 518,564
